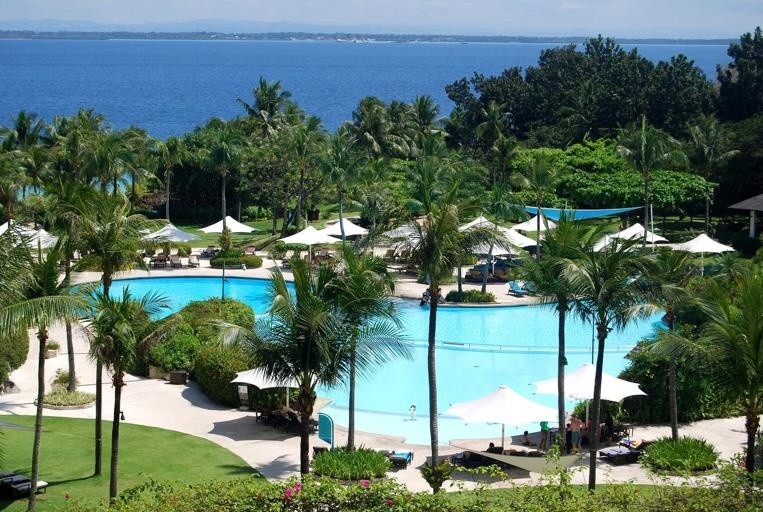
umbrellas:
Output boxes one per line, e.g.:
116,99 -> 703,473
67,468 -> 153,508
230,355 -> 344,410
441,383 -> 571,456
533,362 -> 649,428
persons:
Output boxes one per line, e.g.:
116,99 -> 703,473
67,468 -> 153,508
480,409 -> 613,454
